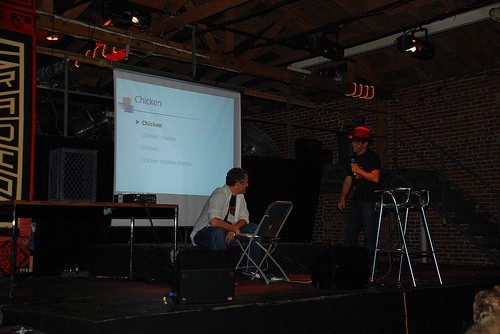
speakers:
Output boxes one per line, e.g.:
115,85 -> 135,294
170,248 -> 236,304
309,246 -> 377,290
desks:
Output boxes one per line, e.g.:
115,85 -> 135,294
0,199 -> 180,299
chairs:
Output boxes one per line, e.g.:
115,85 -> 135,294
236,201 -> 294,285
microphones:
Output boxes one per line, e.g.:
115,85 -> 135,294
351,158 -> 357,175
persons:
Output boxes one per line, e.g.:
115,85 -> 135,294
337,125 -> 381,285
456,286 -> 500,334
190,168 -> 262,278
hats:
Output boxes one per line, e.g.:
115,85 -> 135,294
348,127 -> 370,142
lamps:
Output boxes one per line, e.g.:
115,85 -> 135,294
311,28 -> 344,62
101,0 -> 151,30
396,28 -> 434,61
